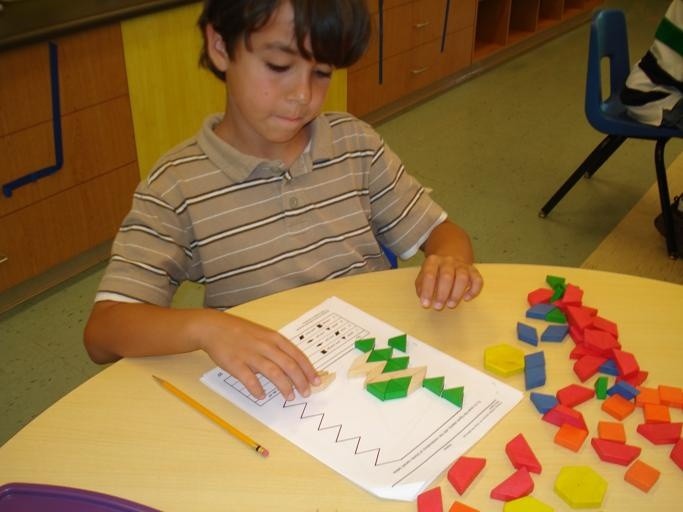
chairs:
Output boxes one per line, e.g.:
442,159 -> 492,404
538,8 -> 683,262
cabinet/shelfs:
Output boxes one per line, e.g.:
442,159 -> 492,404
0,22 -> 142,291
348,0 -> 586,118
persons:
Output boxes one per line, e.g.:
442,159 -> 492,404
620,0 -> 683,257
81,0 -> 484,401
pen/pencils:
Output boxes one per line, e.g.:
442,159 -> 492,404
151,375 -> 269,456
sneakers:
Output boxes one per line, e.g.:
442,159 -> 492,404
654,196 -> 682,258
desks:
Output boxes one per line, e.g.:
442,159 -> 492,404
0,262 -> 683,511
